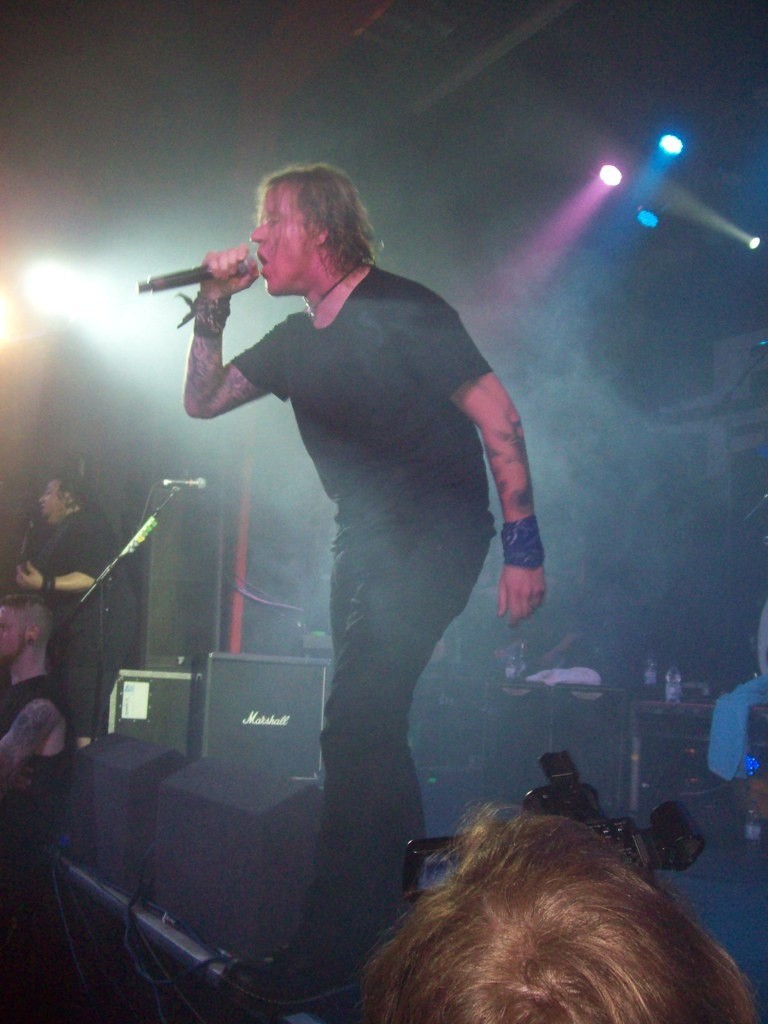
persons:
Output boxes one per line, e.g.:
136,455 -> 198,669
0,592 -> 73,1024
13,474 -> 111,753
351,795 -> 764,1024
183,160 -> 550,1022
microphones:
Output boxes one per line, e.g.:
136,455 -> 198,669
138,256 -> 258,294
162,477 -> 208,491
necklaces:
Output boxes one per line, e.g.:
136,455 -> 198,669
302,257 -> 363,324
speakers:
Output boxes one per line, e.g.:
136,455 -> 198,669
61,650 -> 338,964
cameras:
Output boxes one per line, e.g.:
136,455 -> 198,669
404,751 -> 705,902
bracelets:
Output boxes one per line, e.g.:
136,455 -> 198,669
502,515 -> 546,571
40,573 -> 55,594
179,289 -> 232,337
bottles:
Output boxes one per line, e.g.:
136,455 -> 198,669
644,657 -> 657,687
664,664 -> 682,702
742,809 -> 762,852
